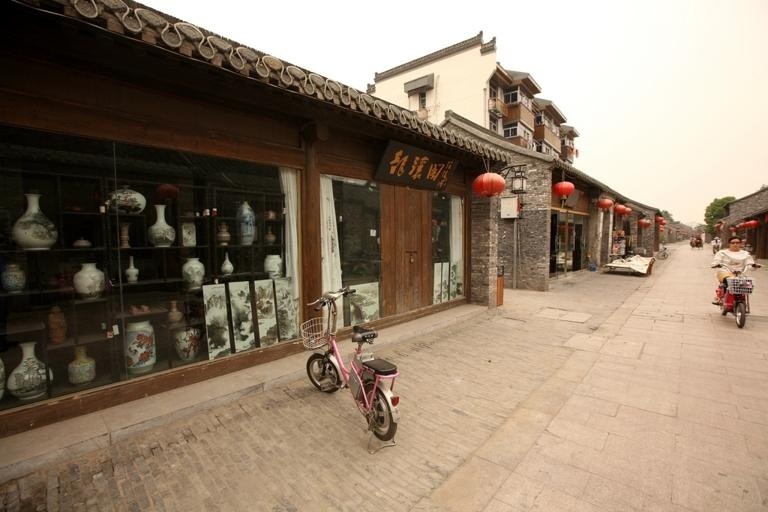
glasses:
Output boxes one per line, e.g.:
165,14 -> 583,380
729,242 -> 742,244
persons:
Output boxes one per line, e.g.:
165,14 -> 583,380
711,237 -> 720,245
711,236 -> 762,305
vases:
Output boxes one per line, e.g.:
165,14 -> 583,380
125,255 -> 140,284
263,255 -> 284,280
217,222 -> 230,246
174,327 -> 201,361
125,320 -> 157,375
265,225 -> 276,246
46,305 -> 69,345
168,300 -> 183,325
8,341 -> 54,401
68,345 -> 96,387
3,263 -> 26,295
236,201 -> 255,246
75,263 -> 104,301
149,203 -> 176,247
118,219 -> 132,248
12,192 -> 59,250
182,256 -> 204,289
106,185 -> 147,215
221,252 -> 234,275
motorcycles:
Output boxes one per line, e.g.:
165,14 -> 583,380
710,263 -> 762,328
300,287 -> 400,454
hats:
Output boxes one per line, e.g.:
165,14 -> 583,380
713,237 -> 720,240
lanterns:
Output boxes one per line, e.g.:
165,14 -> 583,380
597,192 -> 613,209
712,220 -> 759,234
472,157 -> 506,198
656,214 -> 663,222
624,207 -> 632,215
553,169 -> 574,208
615,200 -> 625,214
659,220 -> 667,226
639,216 -> 651,228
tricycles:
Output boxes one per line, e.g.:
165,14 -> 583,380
696,240 -> 703,248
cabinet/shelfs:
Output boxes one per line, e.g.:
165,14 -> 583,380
1,170 -> 116,407
212,181 -> 284,284
104,176 -> 212,381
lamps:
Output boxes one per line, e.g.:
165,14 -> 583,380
499,163 -> 528,195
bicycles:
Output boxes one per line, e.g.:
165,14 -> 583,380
657,247 -> 668,260
